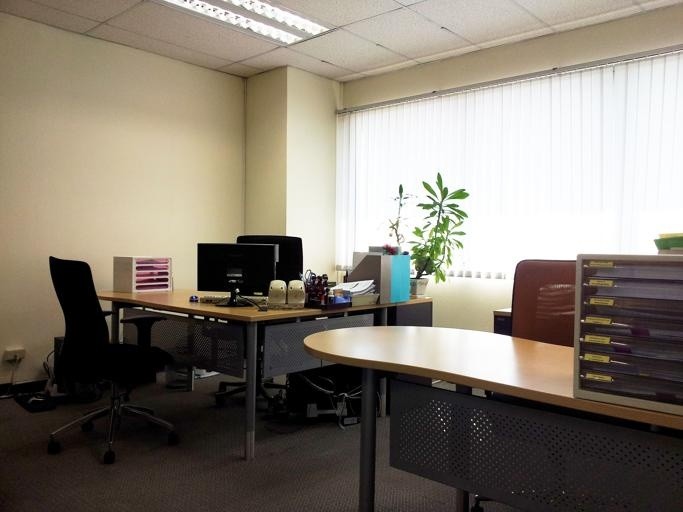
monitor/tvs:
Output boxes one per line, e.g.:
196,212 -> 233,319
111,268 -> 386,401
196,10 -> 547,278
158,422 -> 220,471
237,235 -> 303,287
196,243 -> 277,307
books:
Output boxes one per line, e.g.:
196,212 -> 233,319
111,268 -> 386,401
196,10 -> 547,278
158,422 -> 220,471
329,280 -> 375,297
353,246 -> 401,271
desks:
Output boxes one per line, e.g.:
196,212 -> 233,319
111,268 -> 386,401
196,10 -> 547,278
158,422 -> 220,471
495,308 -> 514,335
304,326 -> 683,511
97,287 -> 398,457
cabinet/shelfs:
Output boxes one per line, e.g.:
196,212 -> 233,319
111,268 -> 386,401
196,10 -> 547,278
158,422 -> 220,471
389,297 -> 435,420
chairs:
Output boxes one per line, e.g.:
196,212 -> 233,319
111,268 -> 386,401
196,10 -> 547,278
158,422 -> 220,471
512,260 -> 576,347
46,256 -> 180,464
215,235 -> 303,413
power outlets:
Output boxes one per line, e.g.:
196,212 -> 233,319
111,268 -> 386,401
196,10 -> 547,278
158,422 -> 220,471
4,349 -> 25,360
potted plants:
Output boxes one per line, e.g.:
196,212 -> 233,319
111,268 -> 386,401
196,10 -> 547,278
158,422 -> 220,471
410,172 -> 469,299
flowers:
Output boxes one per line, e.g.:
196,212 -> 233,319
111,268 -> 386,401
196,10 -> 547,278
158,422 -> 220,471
382,244 -> 397,255
